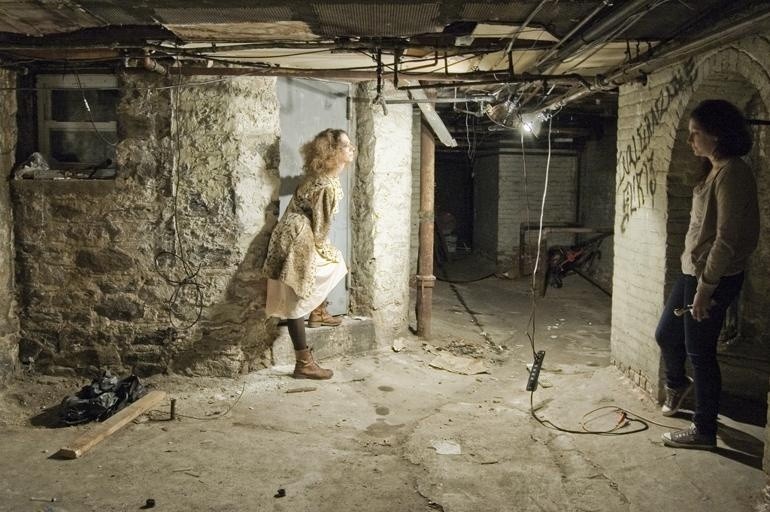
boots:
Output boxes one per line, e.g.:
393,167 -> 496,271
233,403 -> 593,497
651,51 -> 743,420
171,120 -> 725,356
308,298 -> 343,328
293,348 -> 334,379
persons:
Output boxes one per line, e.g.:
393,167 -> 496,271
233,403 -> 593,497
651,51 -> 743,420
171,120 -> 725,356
262,128 -> 356,380
655,99 -> 760,451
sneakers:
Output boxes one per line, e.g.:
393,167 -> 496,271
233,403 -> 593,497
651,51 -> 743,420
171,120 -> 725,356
661,375 -> 694,417
662,422 -> 717,449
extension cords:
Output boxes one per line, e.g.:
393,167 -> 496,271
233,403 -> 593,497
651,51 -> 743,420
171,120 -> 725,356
526,350 -> 545,391
